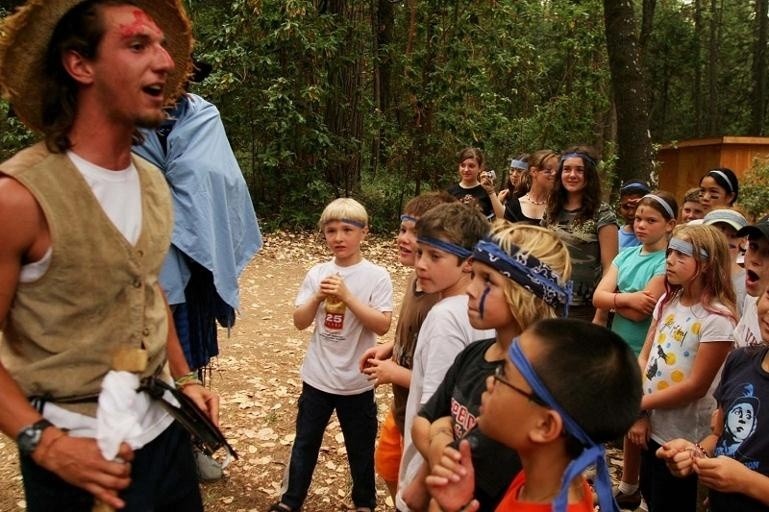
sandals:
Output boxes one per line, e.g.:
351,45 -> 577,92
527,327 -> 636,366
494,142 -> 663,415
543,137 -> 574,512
271,502 -> 297,512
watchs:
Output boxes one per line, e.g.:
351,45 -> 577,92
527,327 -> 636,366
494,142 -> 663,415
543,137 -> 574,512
17,418 -> 56,458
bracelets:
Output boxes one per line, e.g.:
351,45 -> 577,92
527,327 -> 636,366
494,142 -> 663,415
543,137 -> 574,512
37,431 -> 67,467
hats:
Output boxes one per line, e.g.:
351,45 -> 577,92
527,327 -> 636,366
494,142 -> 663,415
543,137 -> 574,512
727,383 -> 760,416
1,0 -> 191,137
734,217 -> 769,243
702,210 -> 747,237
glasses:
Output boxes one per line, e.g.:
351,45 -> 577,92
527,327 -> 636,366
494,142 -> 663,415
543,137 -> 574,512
493,365 -> 548,409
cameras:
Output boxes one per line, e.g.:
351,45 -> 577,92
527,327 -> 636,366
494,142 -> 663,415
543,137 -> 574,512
485,170 -> 497,180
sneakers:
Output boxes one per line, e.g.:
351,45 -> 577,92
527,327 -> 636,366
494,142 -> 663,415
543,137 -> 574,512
612,484 -> 641,511
195,449 -> 223,481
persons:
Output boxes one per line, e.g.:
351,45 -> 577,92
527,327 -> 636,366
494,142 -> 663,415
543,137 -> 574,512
262,196 -> 394,511
360,141 -> 768,511
130,88 -> 265,485
0,0 -> 222,511
